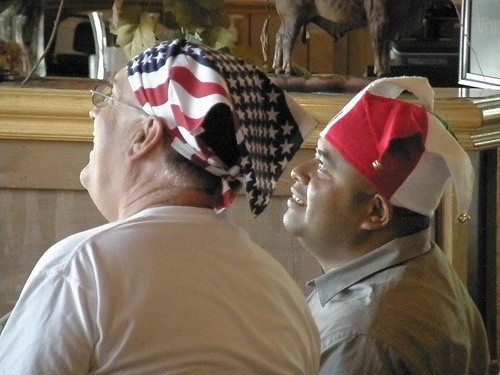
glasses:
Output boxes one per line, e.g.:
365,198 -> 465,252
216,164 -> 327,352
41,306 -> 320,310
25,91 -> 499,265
88,82 -> 150,117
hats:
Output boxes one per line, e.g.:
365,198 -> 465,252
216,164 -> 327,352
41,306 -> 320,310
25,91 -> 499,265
319,75 -> 474,224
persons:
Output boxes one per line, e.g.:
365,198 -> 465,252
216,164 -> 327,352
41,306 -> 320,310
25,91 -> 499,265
282,74 -> 500,375
0,39 -> 321,375
